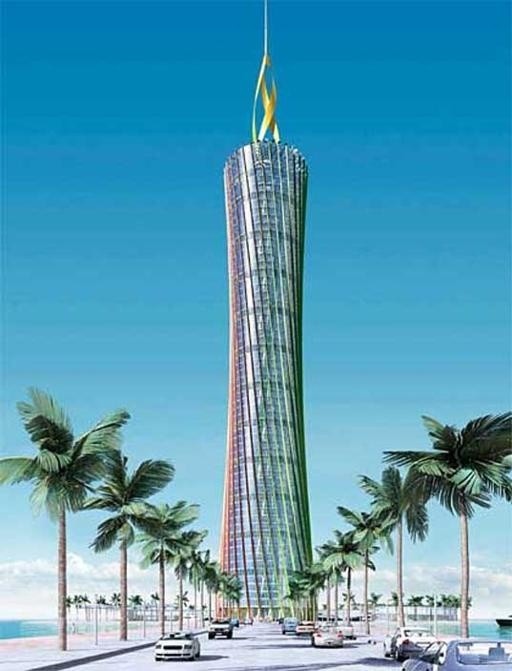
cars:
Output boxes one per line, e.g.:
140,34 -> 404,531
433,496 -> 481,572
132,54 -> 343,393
384,627 -> 512,671
280,613 -> 348,649
152,630 -> 201,663
207,618 -> 233,639
